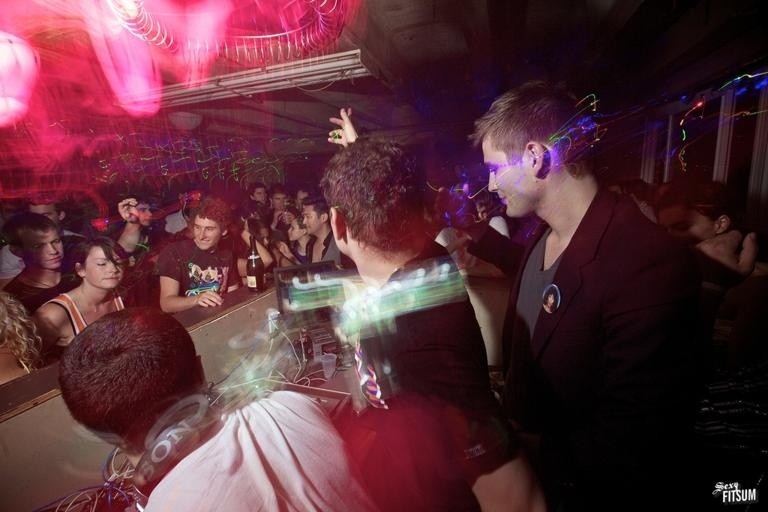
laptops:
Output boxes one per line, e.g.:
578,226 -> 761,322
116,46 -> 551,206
274,260 -> 349,329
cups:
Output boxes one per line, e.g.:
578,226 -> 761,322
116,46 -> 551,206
319,353 -> 337,379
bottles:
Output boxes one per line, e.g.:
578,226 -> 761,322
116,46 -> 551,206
245,233 -> 264,292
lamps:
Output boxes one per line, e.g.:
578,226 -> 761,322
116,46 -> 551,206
111,46 -> 375,114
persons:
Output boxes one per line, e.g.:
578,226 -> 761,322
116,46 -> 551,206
5,79 -> 767,509
